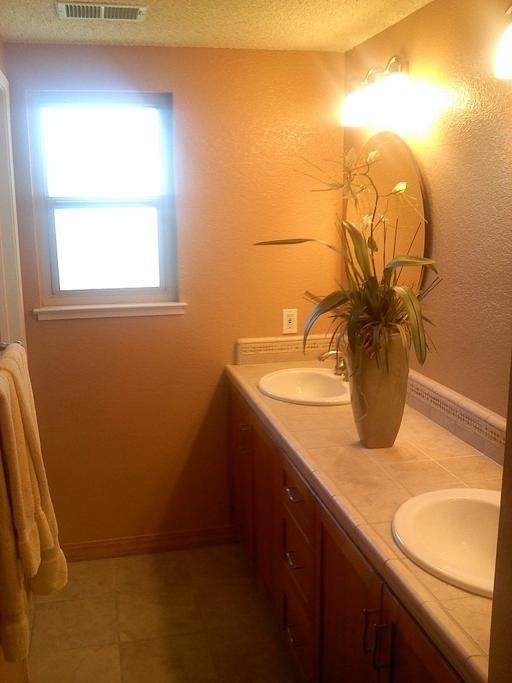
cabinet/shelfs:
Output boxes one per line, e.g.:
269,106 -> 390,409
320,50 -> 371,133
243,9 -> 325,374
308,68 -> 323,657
277,449 -> 321,681
314,501 -> 459,682
227,383 -> 280,616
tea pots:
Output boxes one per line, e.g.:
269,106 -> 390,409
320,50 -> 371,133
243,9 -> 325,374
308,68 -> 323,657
344,131 -> 427,301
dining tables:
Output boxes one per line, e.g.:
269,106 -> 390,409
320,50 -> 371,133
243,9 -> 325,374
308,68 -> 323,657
252,147 -> 444,449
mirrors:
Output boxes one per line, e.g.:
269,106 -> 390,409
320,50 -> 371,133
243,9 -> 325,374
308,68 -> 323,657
344,131 -> 427,301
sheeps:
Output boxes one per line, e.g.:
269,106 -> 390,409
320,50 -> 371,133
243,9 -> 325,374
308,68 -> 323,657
317,349 -> 349,374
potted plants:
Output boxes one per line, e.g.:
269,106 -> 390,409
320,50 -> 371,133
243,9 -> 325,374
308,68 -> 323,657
252,147 -> 444,449
